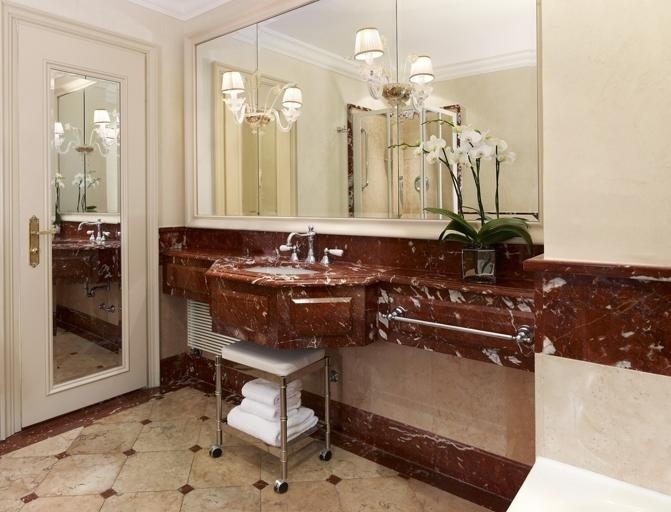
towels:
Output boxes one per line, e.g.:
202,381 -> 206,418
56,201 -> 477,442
227,404 -> 318,446
241,377 -> 304,407
240,397 -> 302,423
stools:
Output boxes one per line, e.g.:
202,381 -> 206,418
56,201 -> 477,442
209,341 -> 332,494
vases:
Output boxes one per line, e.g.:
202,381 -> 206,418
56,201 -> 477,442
460,246 -> 496,285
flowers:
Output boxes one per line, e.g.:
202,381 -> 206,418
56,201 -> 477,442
52,173 -> 65,236
388,118 -> 537,269
72,171 -> 104,213
460,126 -> 531,224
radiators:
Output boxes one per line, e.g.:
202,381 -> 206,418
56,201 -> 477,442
186,299 -> 241,358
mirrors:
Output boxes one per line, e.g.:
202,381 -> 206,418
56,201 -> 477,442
193,0 -> 544,228
45,67 -> 130,395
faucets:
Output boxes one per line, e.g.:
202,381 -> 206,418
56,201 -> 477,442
232,258 -> 331,276
75,218 -> 102,240
284,223 -> 318,265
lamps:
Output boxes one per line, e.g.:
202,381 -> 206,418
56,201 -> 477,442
353,27 -> 398,103
220,71 -> 262,126
92,109 -> 114,158
54,122 -> 80,156
398,55 -> 435,112
258,82 -> 303,133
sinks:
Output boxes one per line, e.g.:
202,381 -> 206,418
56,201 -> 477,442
51,239 -> 79,246
